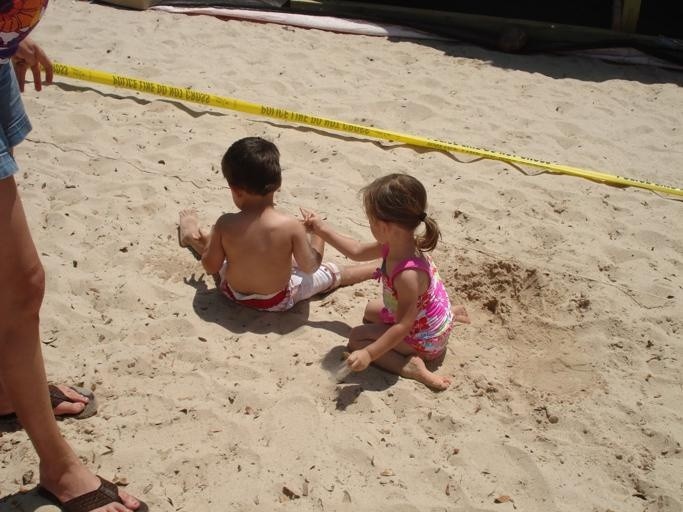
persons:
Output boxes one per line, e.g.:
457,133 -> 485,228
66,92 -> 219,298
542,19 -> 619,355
0,0 -> 152,512
298,173 -> 471,392
177,135 -> 382,314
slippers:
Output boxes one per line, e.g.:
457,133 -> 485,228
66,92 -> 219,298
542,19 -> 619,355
36,470 -> 150,512
0,383 -> 98,423
